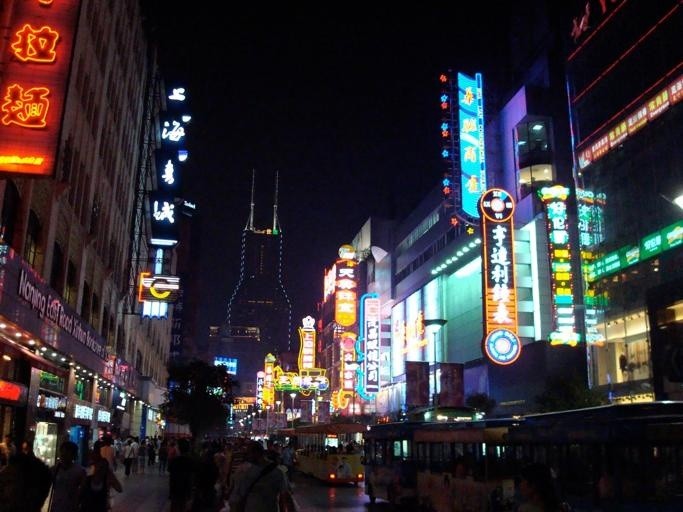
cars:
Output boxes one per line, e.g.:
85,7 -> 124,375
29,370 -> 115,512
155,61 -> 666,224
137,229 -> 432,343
250,426 -> 296,458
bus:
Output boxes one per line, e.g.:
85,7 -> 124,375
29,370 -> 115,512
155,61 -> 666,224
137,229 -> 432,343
360,405 -> 412,508
292,419 -> 365,486
410,409 -> 580,512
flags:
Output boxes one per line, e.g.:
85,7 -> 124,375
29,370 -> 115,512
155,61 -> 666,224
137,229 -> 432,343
438,362 -> 464,407
404,360 -> 430,405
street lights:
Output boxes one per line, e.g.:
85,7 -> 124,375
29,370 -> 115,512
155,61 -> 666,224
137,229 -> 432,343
239,408 -> 262,430
343,359 -> 364,416
310,378 -> 321,424
275,399 -> 281,429
419,319 -> 448,403
265,405 -> 272,432
289,391 -> 297,408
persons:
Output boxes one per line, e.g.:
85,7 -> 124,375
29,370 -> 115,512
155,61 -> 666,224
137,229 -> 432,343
49,441 -> 92,510
92,432 -> 156,480
2,431 -> 52,511
517,463 -> 561,510
594,476 -> 625,510
84,457 -> 123,510
157,432 -> 297,510
